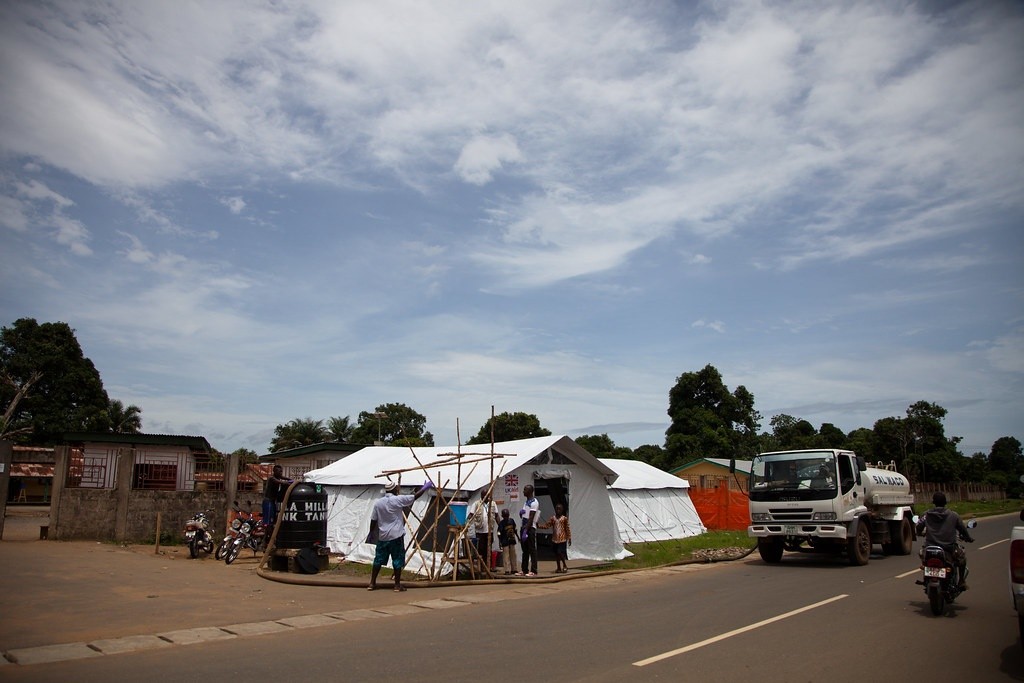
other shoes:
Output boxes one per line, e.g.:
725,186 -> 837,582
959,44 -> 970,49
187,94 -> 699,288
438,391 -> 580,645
556,568 -> 562,573
958,580 -> 970,590
562,566 -> 568,572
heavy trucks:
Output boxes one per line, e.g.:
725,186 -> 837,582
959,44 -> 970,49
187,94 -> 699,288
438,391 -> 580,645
730,448 -> 916,566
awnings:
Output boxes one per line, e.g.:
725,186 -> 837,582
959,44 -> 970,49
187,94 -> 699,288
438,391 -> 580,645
9,464 -> 82,477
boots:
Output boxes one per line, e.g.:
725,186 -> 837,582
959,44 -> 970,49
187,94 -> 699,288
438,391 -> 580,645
393,568 -> 407,592
367,568 -> 379,590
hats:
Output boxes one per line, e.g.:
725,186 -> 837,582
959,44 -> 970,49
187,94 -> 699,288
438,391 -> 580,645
384,480 -> 396,491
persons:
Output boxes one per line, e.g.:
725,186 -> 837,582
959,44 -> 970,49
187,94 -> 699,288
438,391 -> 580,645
810,465 -> 835,489
538,505 -> 572,572
917,493 -> 973,591
515,485 -> 540,577
497,509 -> 519,574
367,479 -> 434,592
261,465 -> 293,543
468,490 -> 500,571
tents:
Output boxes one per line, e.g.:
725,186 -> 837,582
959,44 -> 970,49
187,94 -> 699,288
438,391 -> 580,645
302,435 -> 632,575
595,458 -> 707,543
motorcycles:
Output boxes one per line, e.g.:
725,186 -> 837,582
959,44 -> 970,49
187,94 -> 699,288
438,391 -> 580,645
183,508 -> 215,558
911,514 -> 980,617
226,500 -> 267,563
215,500 -> 249,560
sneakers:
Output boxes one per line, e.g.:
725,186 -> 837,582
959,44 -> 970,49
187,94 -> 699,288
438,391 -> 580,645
515,571 -> 525,576
525,572 -> 537,576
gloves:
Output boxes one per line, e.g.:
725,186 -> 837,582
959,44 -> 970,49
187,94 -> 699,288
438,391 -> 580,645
422,481 -> 432,490
519,509 -> 526,516
522,528 -> 528,541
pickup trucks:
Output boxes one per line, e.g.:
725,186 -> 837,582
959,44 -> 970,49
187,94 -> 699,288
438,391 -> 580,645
1008,507 -> 1024,640
